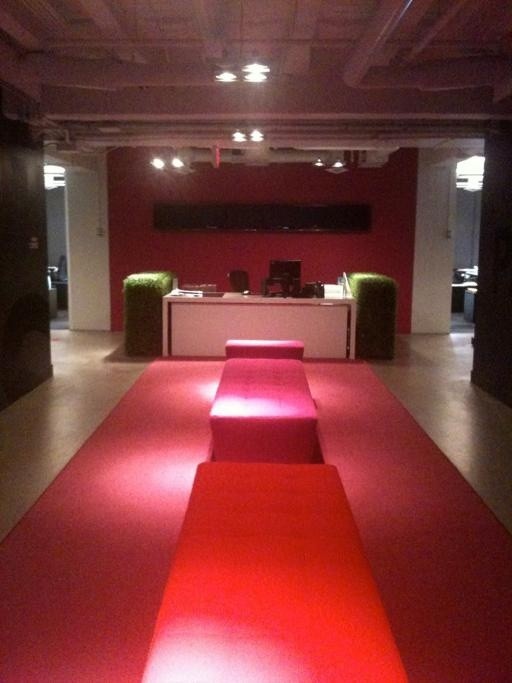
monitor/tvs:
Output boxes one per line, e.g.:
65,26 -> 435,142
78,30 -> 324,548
270,260 -> 301,279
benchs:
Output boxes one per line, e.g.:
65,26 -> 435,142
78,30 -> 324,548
207,357 -> 325,464
224,338 -> 305,361
141,461 -> 408,683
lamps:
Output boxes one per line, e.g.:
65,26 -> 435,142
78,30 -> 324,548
148,154 -> 189,172
231,128 -> 264,143
206,47 -> 277,84
312,158 -> 346,170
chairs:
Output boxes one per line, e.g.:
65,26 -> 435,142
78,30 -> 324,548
227,270 -> 249,292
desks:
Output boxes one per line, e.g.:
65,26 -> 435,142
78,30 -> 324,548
160,288 -> 358,359
